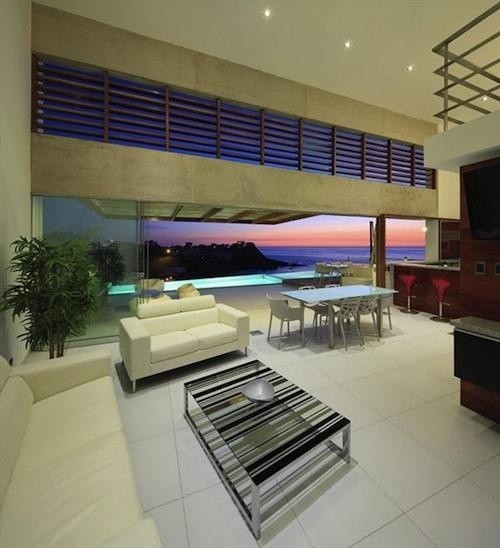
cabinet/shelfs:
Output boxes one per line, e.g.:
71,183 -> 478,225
451,316 -> 500,425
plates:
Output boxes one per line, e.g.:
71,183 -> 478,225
240,378 -> 277,403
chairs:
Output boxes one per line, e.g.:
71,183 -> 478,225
358,305 -> 393,331
319,295 -> 362,350
319,284 -> 347,327
397,273 -> 418,314
315,263 -> 325,281
430,277 -> 453,324
319,265 -> 332,283
266,293 -> 305,350
298,285 -> 334,337
342,294 -> 381,344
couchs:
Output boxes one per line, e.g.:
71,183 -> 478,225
0,347 -> 162,548
119,292 -> 250,393
129,289 -> 171,313
177,283 -> 200,299
135,278 -> 164,291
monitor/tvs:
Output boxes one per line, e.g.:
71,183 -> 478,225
463,164 -> 500,241
